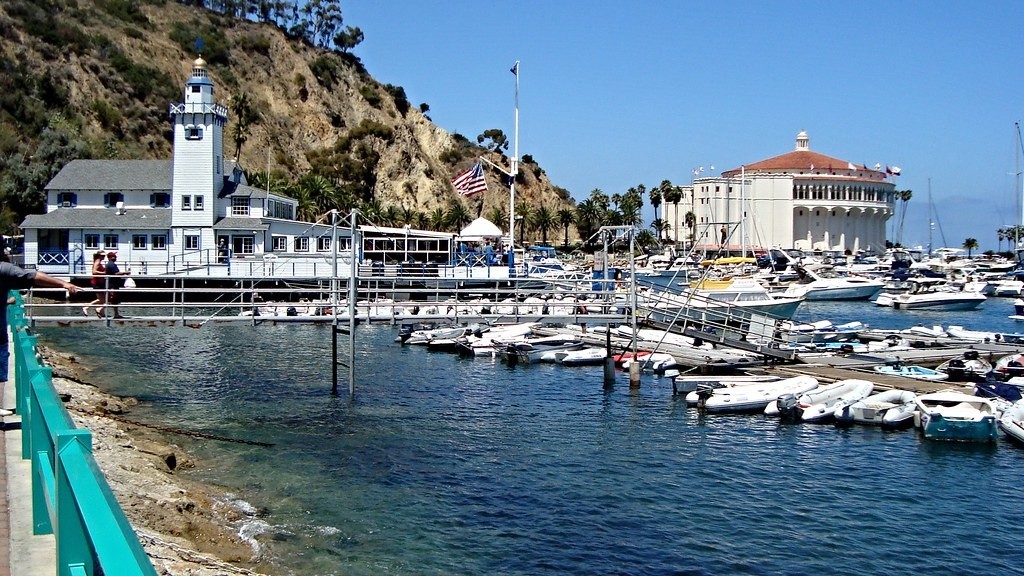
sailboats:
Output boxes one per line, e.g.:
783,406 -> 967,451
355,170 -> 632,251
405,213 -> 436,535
639,163 -> 806,328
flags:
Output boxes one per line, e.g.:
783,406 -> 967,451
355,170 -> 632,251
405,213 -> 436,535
451,160 -> 489,198
691,165 -> 704,176
847,163 -> 901,177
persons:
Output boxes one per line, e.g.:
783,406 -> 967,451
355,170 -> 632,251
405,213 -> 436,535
459,241 -> 506,266
82,250 -> 131,319
720,226 -> 727,244
216,238 -> 227,263
0,232 -> 84,416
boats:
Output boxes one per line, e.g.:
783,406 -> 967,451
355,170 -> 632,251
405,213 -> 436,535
916,389 -> 1000,444
239,242 -> 1024,364
785,265 -> 887,301
852,389 -> 919,426
763,378 -> 873,422
621,352 -> 678,374
684,376 -> 820,414
893,277 -> 988,311
869,281 -> 905,307
990,353 -> 1024,380
873,363 -> 950,382
611,351 -> 651,365
935,351 -> 992,377
1001,398 -> 1023,442
665,370 -> 780,394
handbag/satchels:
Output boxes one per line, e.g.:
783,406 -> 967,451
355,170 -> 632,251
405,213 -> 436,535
124,278 -> 136,288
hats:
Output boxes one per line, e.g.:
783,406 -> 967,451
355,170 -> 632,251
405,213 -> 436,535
107,251 -> 117,258
97,250 -> 106,256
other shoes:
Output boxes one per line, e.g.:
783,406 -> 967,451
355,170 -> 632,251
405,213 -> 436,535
95,308 -> 101,319
0,407 -> 13,416
114,314 -> 124,319
81,307 -> 88,316
